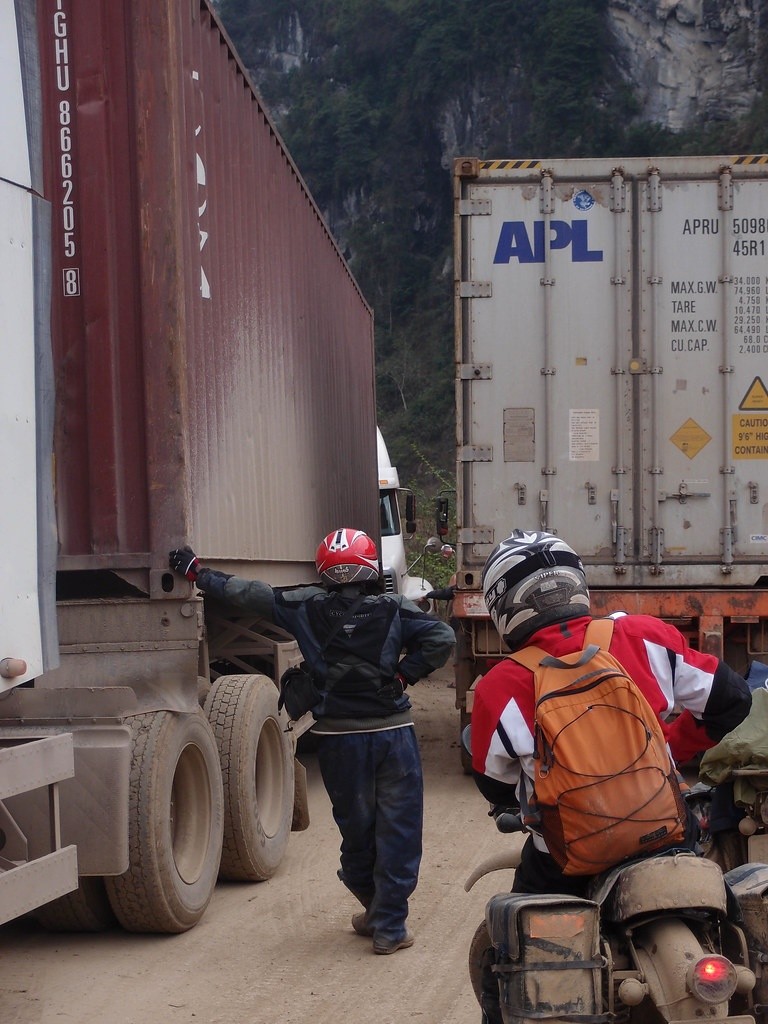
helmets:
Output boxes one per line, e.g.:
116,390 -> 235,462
483,528 -> 589,652
315,527 -> 379,587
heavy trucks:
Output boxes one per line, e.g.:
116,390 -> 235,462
434,151 -> 768,773
377,430 -> 448,620
1,1 -> 379,941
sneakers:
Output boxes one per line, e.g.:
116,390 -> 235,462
351,912 -> 373,936
373,929 -> 415,954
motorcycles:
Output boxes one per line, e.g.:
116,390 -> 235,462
463,724 -> 768,1024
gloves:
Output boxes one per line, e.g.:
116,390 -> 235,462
168,545 -> 202,581
377,673 -> 407,701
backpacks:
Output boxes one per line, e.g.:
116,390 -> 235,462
503,617 -> 687,879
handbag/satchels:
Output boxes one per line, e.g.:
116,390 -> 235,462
277,666 -> 321,722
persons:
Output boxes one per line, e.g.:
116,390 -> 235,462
471,530 -> 753,899
168,527 -> 455,954
